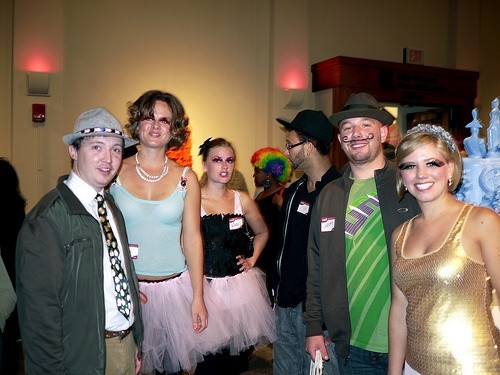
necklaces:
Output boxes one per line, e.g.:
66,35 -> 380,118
133,152 -> 171,184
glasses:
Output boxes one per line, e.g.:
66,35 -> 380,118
285,138 -> 313,150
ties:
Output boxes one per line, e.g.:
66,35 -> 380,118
95,194 -> 132,321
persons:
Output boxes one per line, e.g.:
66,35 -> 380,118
305,92 -> 421,375
386,124 -> 500,374
274,109 -> 344,375
16,108 -> 144,375
180,137 -> 277,374
247,147 -> 293,309
0,253 -> 17,332
104,90 -> 208,374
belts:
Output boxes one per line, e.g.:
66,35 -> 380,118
104,326 -> 133,340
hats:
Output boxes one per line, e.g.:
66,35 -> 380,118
276,109 -> 335,143
329,92 -> 396,128
62,107 -> 140,149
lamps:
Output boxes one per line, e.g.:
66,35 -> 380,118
281,87 -> 306,110
25,70 -> 54,99
